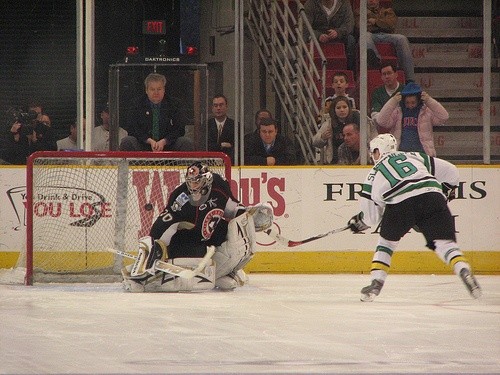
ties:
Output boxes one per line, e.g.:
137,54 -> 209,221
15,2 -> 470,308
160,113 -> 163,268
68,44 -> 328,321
217,124 -> 223,144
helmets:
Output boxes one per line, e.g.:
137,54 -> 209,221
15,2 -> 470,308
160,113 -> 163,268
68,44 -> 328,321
368,133 -> 397,164
182,161 -> 213,194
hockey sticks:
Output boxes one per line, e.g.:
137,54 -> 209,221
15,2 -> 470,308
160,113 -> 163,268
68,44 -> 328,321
266,224 -> 351,248
106,244 -> 216,280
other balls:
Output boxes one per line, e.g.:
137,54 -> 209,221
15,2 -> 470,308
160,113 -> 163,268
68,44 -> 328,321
145,204 -> 152,210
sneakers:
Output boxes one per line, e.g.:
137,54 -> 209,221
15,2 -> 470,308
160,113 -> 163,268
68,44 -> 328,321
460,267 -> 483,301
360,277 -> 385,302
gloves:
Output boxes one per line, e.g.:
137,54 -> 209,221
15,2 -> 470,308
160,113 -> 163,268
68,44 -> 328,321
348,211 -> 371,234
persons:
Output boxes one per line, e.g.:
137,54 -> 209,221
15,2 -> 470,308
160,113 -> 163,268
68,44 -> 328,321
376,81 -> 449,158
299,0 -> 414,85
147,161 -> 274,268
353,133 -> 481,303
57,106 -> 128,152
202,95 -> 241,165
312,72 -> 372,165
372,64 -> 406,117
120,73 -> 195,154
0,106 -> 57,164
238,110 -> 299,165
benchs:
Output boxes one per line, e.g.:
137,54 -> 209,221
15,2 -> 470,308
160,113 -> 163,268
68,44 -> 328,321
305,42 -> 405,96
